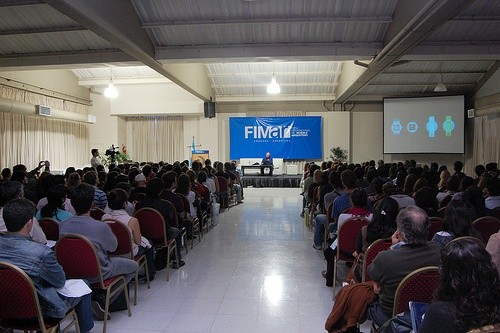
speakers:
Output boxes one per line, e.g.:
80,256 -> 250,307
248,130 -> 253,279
204,101 -> 215,118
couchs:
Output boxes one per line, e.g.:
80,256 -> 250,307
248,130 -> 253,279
240,158 -> 284,176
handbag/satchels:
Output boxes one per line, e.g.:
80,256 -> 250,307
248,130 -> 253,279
212,195 -> 220,225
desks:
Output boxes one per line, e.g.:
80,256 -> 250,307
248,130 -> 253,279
286,165 -> 299,175
241,165 -> 272,176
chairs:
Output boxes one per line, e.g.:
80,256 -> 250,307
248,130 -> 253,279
302,171 -> 500,333
0,172 -> 240,333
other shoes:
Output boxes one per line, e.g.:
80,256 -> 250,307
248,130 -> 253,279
322,270 -> 327,278
173,260 -> 185,268
299,213 -> 304,218
91,301 -> 111,320
312,244 -> 323,250
238,201 -> 243,203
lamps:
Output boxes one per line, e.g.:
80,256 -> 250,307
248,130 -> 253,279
266,64 -> 280,93
103,68 -> 118,98
433,75 -> 447,92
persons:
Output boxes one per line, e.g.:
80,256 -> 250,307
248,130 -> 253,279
91,149 -> 99,171
0,161 -> 244,333
259,153 -> 274,176
111,144 -> 127,162
300,158 -> 500,333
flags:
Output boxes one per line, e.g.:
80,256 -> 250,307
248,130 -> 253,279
192,139 -> 195,149
